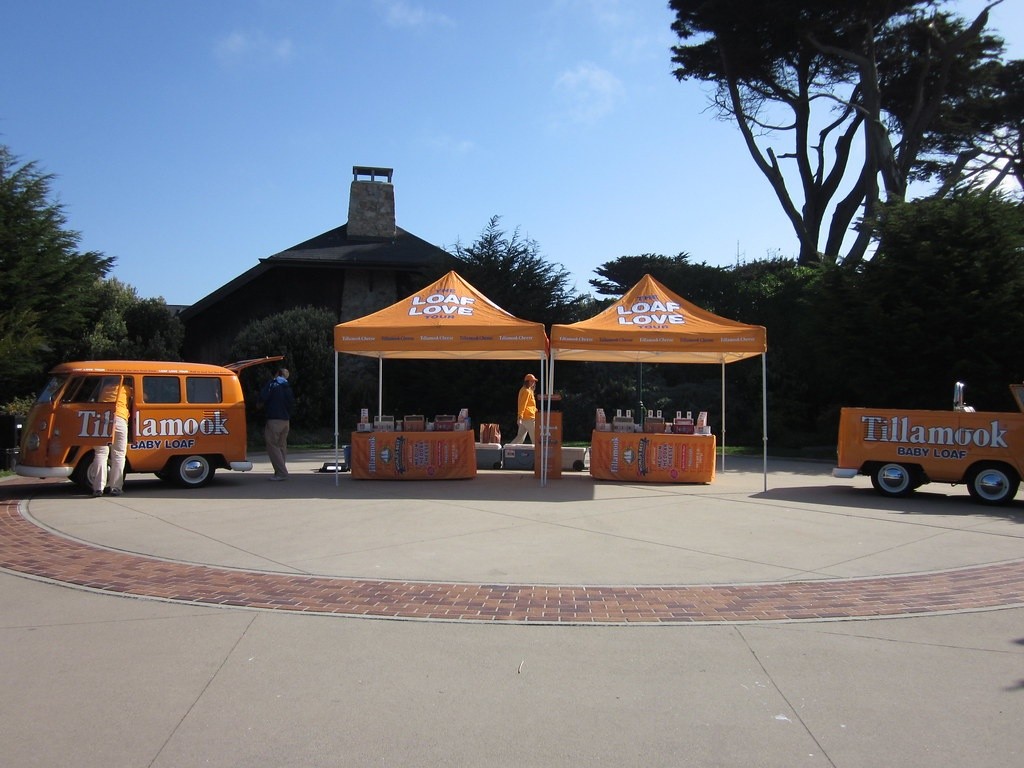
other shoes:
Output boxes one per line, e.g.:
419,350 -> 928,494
110,488 -> 122,495
270,476 -> 288,480
94,490 -> 103,497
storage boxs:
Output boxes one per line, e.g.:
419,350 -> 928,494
434,415 -> 455,431
699,426 -> 711,433
475,442 -> 501,470
644,417 -> 665,433
374,416 -> 393,431
404,416 -> 424,432
454,423 -> 465,430
356,422 -> 373,432
503,444 -> 534,470
673,418 -> 695,434
563,447 -> 588,471
342,444 -> 352,467
613,416 -> 634,432
597,423 -> 612,432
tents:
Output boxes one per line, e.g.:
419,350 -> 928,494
543,273 -> 768,493
334,271 -> 545,489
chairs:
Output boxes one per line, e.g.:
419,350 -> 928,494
952,381 -> 977,413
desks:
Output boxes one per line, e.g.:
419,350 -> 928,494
350,430 -> 476,480
590,430 -> 717,486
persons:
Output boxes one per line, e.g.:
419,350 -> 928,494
510,374 -> 539,445
92,382 -> 134,498
255,368 -> 290,482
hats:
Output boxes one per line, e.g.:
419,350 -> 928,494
525,374 -> 537,381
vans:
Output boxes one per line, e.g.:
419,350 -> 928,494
17,356 -> 285,490
